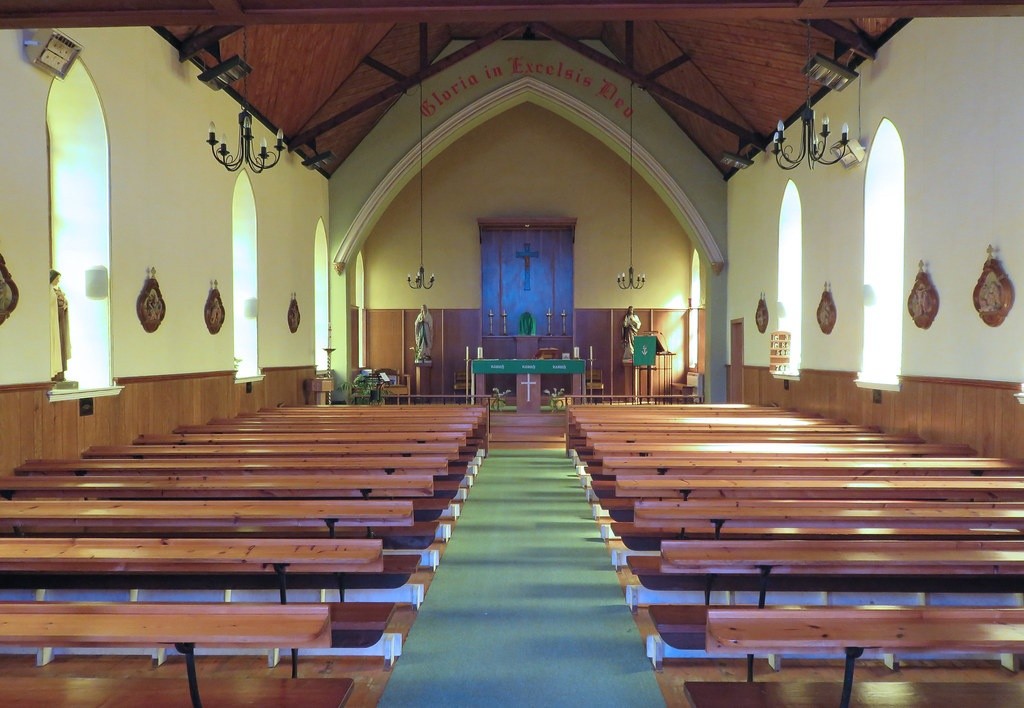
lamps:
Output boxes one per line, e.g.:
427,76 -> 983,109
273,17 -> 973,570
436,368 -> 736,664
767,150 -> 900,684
300,140 -> 336,171
197,45 -> 256,91
207,25 -> 287,174
771,18 -> 851,171
718,138 -> 755,169
834,139 -> 866,170
590,346 -> 592,360
407,84 -> 435,289
802,37 -> 859,94
617,80 -> 645,291
22,28 -> 85,83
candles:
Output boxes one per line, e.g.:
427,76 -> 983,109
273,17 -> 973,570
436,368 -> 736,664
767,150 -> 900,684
574,347 -> 579,358
478,347 -> 483,359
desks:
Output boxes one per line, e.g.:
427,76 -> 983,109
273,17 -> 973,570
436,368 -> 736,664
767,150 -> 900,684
471,359 -> 586,413
3,403 -> 486,649
566,405 -> 1024,658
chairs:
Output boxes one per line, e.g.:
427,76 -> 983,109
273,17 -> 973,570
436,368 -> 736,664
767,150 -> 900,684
375,368 -> 411,404
454,371 -> 471,395
587,369 -> 603,402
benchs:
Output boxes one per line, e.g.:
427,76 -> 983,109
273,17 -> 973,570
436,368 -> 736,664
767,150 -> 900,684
0,432 -> 489,707
568,426 -> 1023,708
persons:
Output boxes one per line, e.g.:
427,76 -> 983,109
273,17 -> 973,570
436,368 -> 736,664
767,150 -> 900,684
623,306 -> 641,359
415,304 -> 434,359
49,269 -> 69,382
